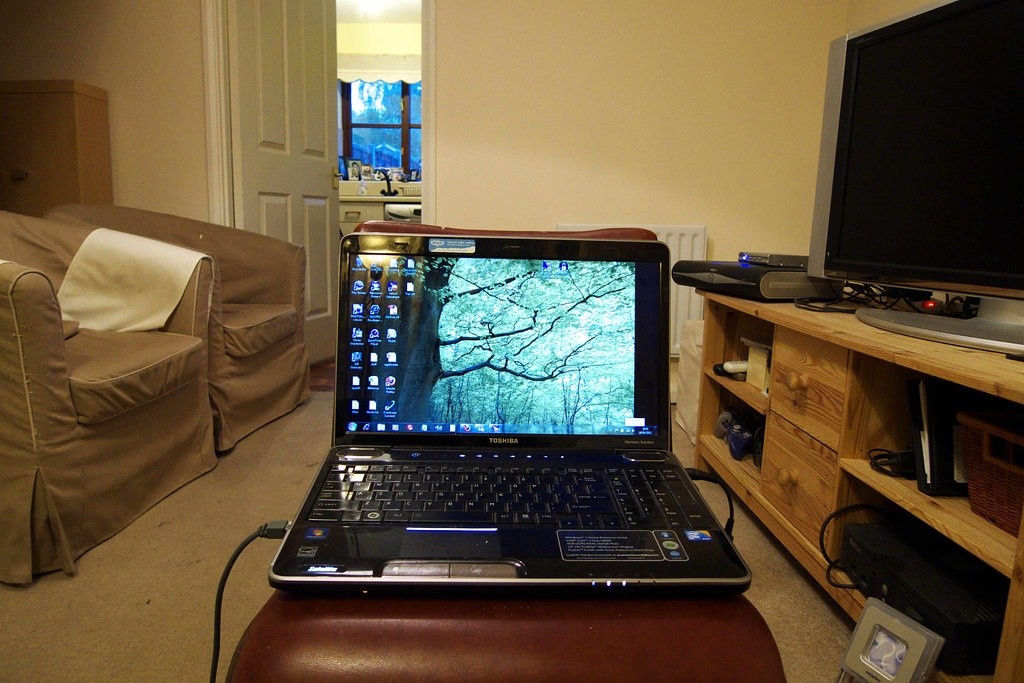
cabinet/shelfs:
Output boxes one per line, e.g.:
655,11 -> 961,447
696,287 -> 1024,683
0,80 -> 113,216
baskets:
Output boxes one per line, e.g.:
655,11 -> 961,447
956,407 -> 1024,538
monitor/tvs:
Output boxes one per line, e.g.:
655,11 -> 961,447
807,0 -> 1023,358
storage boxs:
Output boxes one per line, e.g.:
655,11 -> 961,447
957,411 -> 1024,539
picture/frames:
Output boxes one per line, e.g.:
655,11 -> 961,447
339,202 -> 385,299
346,157 -> 362,181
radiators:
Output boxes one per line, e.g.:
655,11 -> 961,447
557,224 -> 707,357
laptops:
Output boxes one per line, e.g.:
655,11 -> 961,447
266,232 -> 753,600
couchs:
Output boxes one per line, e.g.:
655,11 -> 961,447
42,202 -> 306,455
0,210 -> 219,587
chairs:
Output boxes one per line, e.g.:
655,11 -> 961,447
223,218 -> 789,683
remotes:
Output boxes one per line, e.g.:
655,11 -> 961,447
712,360 -> 748,381
